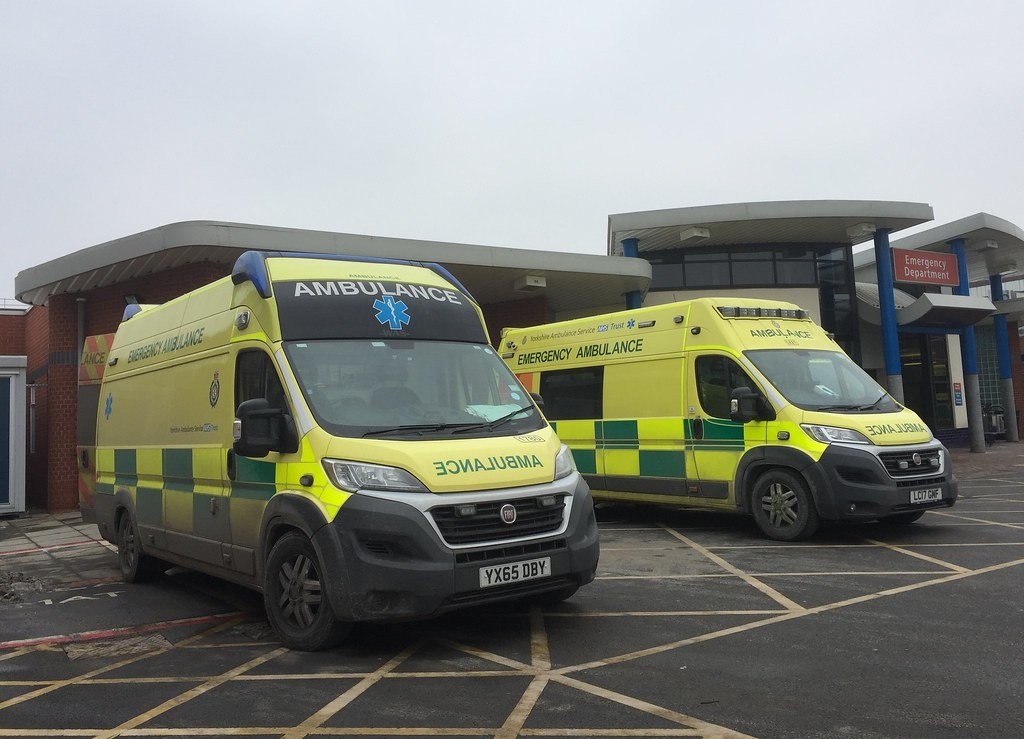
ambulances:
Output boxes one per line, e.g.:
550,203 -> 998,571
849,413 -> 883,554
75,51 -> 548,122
75,247 -> 603,652
487,296 -> 959,541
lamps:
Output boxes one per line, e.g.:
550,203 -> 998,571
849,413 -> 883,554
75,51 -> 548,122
997,264 -> 1019,276
846,223 -> 877,238
513,275 -> 547,295
974,240 -> 1000,253
681,228 -> 708,244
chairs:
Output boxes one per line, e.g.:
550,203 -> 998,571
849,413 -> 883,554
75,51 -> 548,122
363,359 -> 424,413
280,358 -> 341,426
